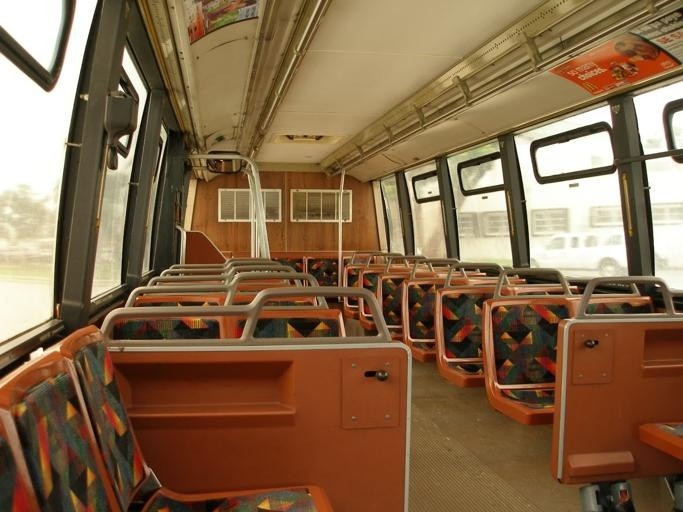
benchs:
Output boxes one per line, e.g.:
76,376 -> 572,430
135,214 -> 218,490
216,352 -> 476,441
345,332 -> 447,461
0,253 -> 682,512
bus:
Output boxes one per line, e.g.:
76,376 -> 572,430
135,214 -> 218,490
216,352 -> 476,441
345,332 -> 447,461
408,82 -> 683,278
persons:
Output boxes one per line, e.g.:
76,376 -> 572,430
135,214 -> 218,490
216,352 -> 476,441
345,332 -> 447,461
613,41 -> 658,61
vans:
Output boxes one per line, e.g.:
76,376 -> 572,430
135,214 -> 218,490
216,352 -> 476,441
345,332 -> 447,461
530,228 -> 626,272
